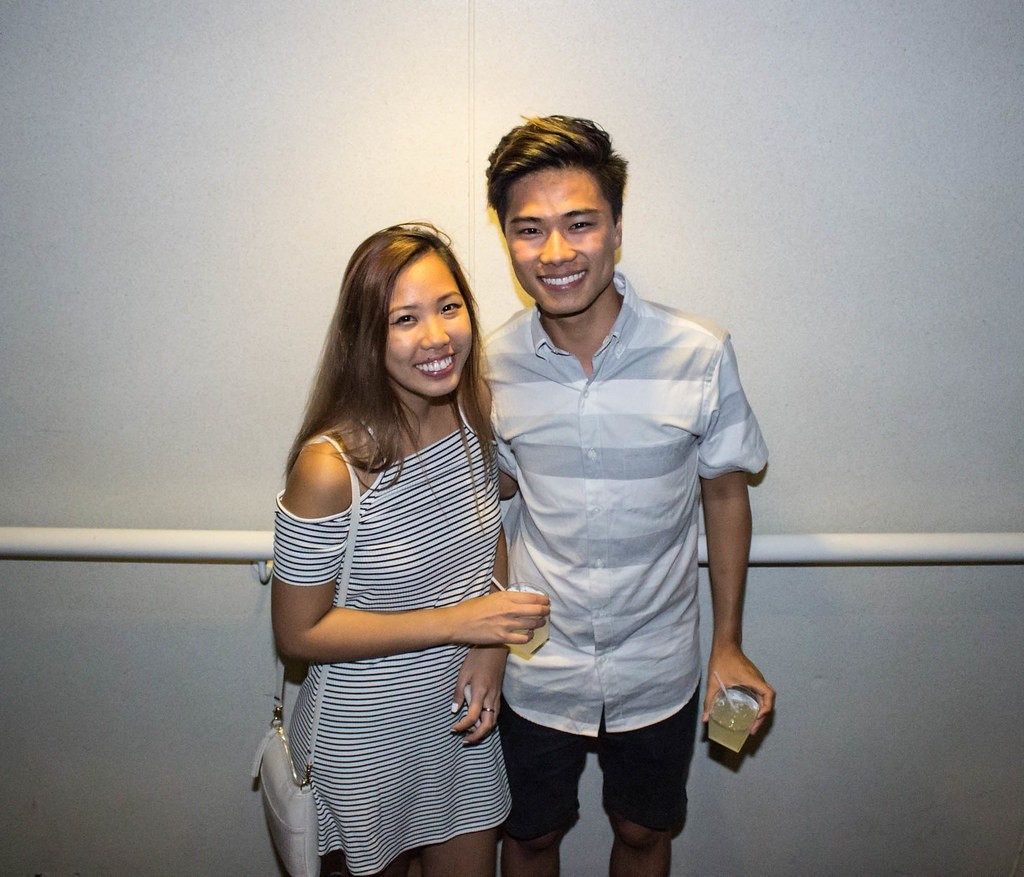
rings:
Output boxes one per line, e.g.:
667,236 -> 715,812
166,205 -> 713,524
482,708 -> 495,713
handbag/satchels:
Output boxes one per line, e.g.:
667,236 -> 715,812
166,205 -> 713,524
250,704 -> 321,877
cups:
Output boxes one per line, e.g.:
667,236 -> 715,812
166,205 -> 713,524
502,583 -> 550,657
706,687 -> 761,753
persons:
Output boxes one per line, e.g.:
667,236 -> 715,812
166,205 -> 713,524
273,221 -> 551,877
479,116 -> 775,877
